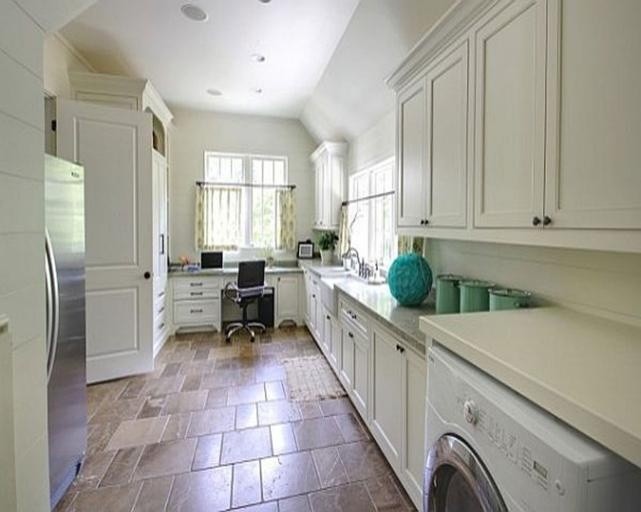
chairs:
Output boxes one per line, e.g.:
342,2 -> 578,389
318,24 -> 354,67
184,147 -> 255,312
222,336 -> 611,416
225,261 -> 267,343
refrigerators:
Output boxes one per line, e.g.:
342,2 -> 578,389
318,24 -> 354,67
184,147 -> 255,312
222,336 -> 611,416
41,152 -> 87,512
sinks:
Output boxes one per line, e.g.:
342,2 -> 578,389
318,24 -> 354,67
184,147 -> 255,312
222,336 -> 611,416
320,277 -> 360,313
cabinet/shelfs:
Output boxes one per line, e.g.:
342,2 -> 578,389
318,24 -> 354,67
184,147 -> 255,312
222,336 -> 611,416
386,2 -> 640,250
165,264 -> 305,338
65,70 -> 174,358
297,251 -> 640,511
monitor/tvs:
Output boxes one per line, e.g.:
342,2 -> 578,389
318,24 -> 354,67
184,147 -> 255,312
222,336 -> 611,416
201,251 -> 223,269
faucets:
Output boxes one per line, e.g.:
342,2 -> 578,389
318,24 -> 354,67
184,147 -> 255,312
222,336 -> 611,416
345,247 -> 360,266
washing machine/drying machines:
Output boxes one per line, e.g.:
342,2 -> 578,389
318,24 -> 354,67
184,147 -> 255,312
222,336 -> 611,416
422,343 -> 641,512
